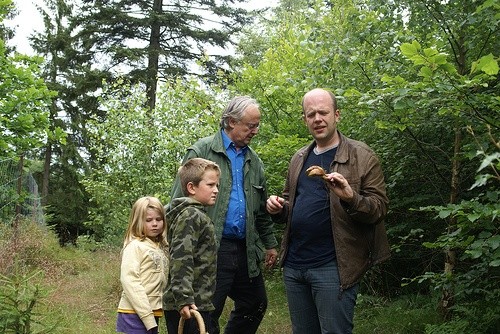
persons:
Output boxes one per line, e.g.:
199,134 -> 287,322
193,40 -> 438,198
167,94 -> 279,334
115,196 -> 171,334
265,86 -> 389,334
162,158 -> 223,334
